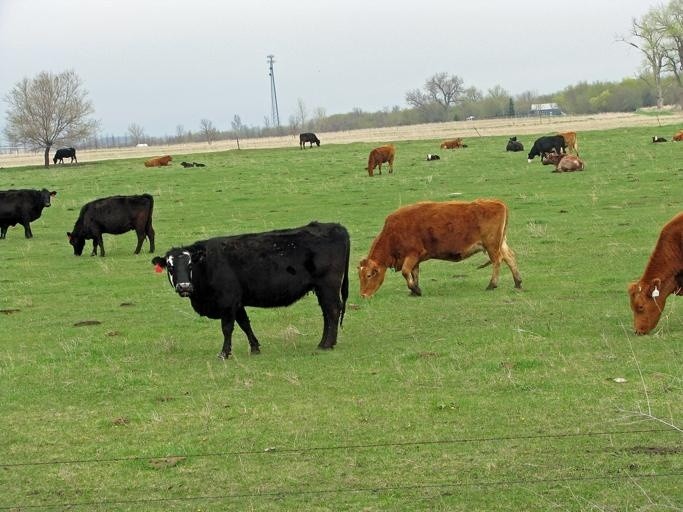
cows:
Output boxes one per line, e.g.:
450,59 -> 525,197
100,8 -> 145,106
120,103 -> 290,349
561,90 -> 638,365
53,147 -> 78,166
180,161 -> 205,168
627,211 -> 683,336
356,198 -> 523,300
299,132 -> 321,150
0,188 -> 56,240
151,220 -> 351,360
526,131 -> 584,172
66,193 -> 156,257
365,144 -> 396,176
651,129 -> 683,143
144,154 -> 175,167
426,152 -> 440,161
506,136 -> 524,152
440,137 -> 467,150
